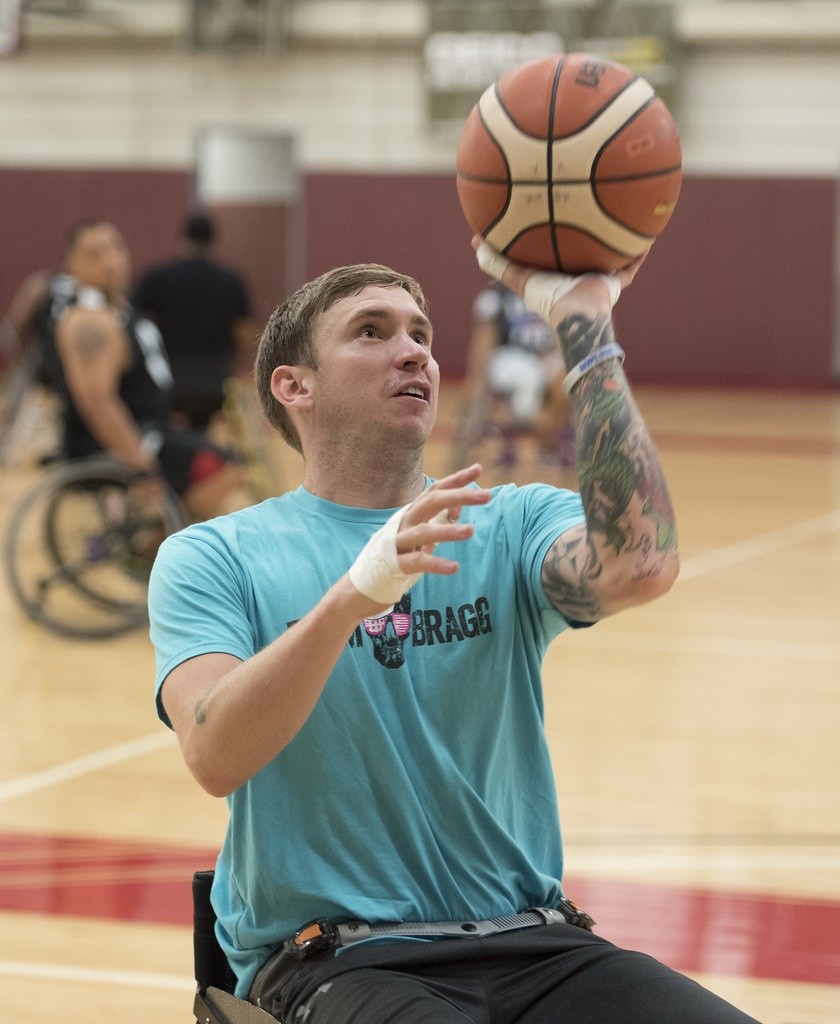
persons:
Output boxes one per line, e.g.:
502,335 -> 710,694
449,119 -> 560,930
468,272 -> 578,464
1,210 -> 275,556
146,234 -> 770,1023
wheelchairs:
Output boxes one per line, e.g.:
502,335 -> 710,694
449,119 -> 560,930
438,379 -> 579,471
0,328 -> 272,645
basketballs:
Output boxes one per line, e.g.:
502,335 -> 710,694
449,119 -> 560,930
455,52 -> 682,275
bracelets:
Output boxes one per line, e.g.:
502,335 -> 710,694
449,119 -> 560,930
561,341 -> 626,400
